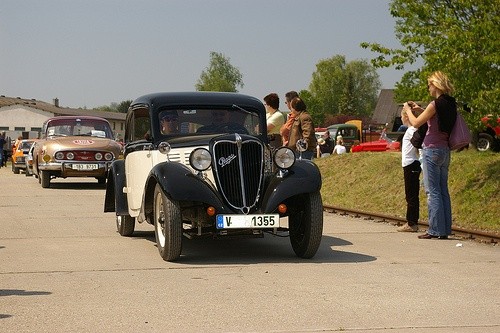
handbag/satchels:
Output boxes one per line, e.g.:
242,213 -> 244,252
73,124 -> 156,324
449,109 -> 470,150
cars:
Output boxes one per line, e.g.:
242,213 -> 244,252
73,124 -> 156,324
104,92 -> 323,261
11,116 -> 124,188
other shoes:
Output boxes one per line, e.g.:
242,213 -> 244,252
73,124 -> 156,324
396,221 -> 417,232
418,232 -> 448,239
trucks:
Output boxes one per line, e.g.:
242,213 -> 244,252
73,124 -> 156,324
327,120 -> 404,147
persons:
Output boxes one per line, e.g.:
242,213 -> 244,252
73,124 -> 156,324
159,110 -> 180,134
286,96 -> 318,160
0,130 -> 31,167
196,110 -> 251,135
316,117 -> 408,158
263,92 -> 285,149
396,105 -> 429,233
279,91 -> 300,146
402,70 -> 457,241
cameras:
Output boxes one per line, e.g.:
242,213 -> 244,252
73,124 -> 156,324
408,104 -> 412,109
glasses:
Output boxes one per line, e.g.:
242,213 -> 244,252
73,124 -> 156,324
164,115 -> 177,121
285,101 -> 287,104
428,82 -> 432,88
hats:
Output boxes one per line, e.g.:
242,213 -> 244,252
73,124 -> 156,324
160,109 -> 179,118
